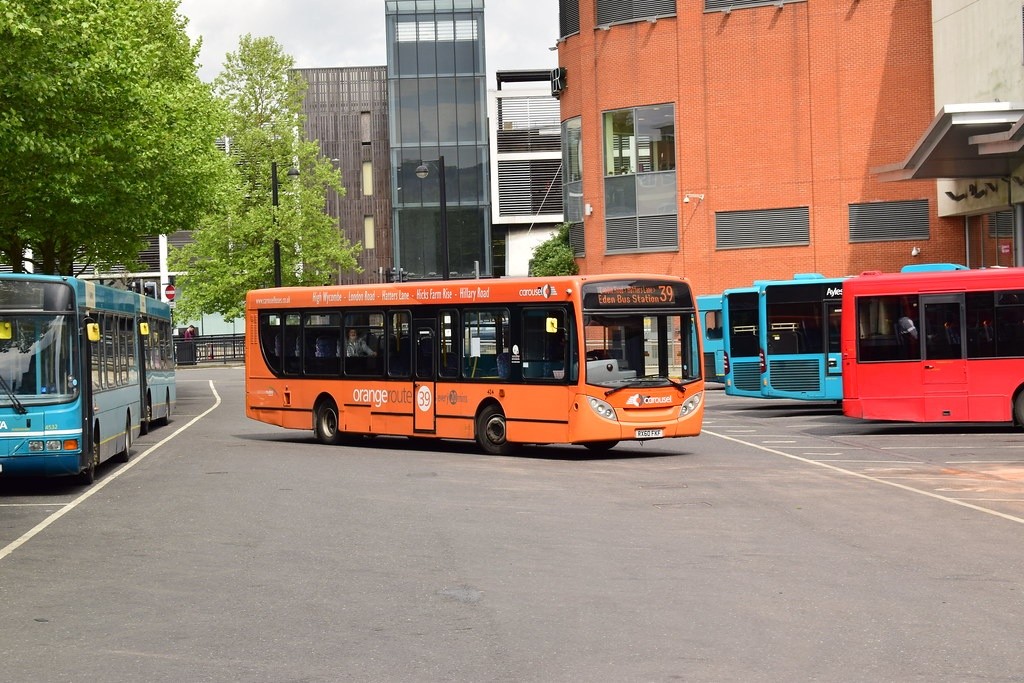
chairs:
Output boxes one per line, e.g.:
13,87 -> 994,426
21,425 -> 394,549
891,322 -> 996,353
269,327 -> 514,381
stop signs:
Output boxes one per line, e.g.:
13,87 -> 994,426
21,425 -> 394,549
165,285 -> 176,301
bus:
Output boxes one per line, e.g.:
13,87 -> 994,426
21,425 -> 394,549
689,278 -> 774,391
244,273 -> 706,459
720,272 -> 825,400
0,272 -> 178,487
841,266 -> 1024,428
754,262 -> 971,406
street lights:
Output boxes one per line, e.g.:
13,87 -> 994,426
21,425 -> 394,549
270,160 -> 300,291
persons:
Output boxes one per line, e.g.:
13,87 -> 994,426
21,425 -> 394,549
184,325 -> 196,342
347,328 -> 378,357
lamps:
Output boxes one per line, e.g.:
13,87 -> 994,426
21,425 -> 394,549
774,0 -> 785,8
549,47 -> 558,50
986,182 -> 997,193
647,14 -> 659,24
601,21 -> 616,30
945,191 -> 966,202
1000,176 -> 1010,183
969,184 -> 985,199
721,4 -> 733,13
1010,176 -> 1023,186
558,38 -> 566,42
